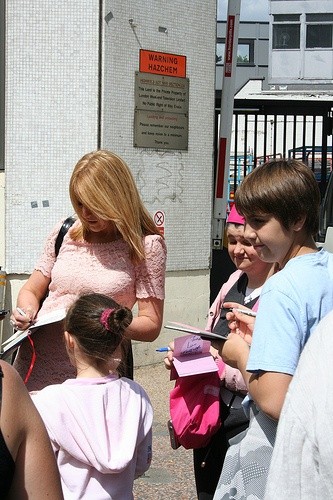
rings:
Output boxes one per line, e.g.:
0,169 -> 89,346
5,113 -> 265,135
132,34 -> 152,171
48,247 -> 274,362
12,320 -> 16,326
16,326 -> 19,330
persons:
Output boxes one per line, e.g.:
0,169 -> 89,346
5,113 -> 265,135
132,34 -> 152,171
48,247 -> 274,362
1,358 -> 64,500
27,293 -> 153,500
209,159 -> 333,500
164,204 -> 278,500
5,150 -> 166,392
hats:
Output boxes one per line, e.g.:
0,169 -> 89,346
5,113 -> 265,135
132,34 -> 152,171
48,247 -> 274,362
226,197 -> 245,225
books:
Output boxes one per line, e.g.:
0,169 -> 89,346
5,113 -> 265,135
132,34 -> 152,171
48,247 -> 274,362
170,334 -> 219,381
2,307 -> 68,353
164,321 -> 228,340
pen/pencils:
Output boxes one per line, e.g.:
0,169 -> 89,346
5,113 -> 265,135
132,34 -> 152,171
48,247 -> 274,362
155,347 -> 172,353
16,308 -> 34,325
219,307 -> 256,317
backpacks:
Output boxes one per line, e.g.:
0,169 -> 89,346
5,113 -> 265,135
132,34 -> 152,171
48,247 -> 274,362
167,274 -> 249,467
